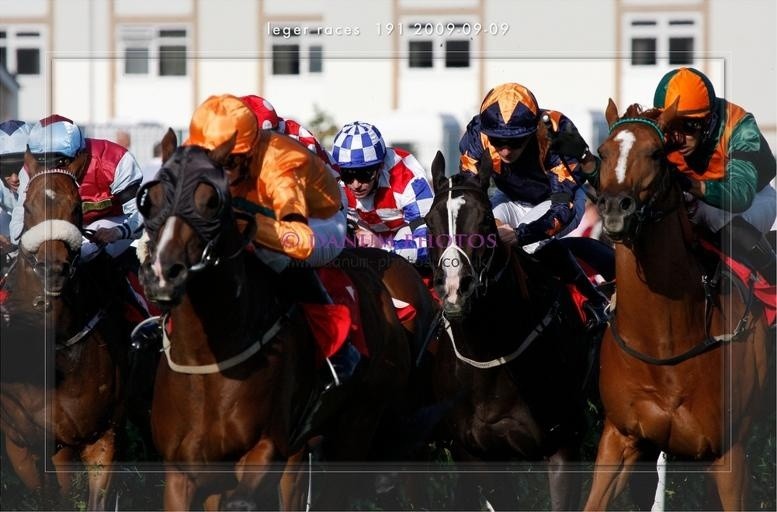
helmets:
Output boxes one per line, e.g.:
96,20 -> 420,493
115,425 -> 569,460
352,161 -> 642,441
238,95 -> 279,130
331,122 -> 386,166
1,120 -> 30,155
182,94 -> 259,153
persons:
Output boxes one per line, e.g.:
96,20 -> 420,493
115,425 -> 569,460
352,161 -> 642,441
459,82 -> 601,256
0,120 -> 36,247
239,94 -> 348,218
328,122 -> 434,268
113,126 -> 139,159
8,114 -> 167,450
604,65 -> 776,315
136,93 -> 347,268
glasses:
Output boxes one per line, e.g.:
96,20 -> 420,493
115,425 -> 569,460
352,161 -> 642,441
27,114 -> 85,157
35,157 -> 69,168
340,168 -> 377,183
1,161 -> 23,177
673,120 -> 711,138
488,136 -> 527,149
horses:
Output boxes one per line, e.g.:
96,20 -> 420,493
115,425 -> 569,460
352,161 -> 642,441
139,127 -> 436,511
424,147 -> 616,511
0,143 -> 160,512
580,97 -> 776,511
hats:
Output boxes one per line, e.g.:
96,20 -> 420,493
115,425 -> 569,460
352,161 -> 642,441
479,83 -> 539,138
654,68 -> 715,118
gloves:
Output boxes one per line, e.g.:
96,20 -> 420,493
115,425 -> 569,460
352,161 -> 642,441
90,227 -> 121,243
